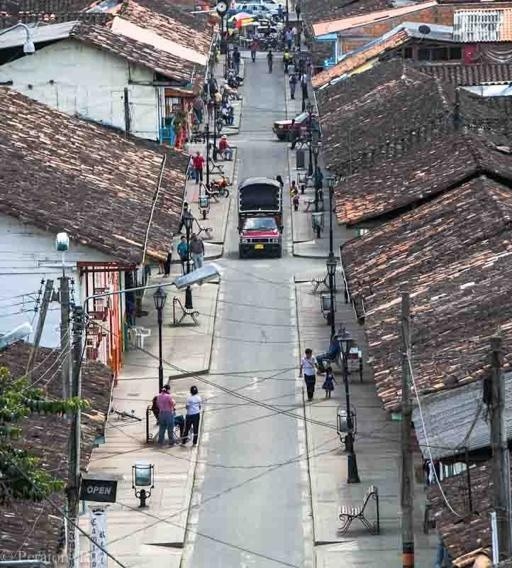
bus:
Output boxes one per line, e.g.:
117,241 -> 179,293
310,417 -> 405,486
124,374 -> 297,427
237,176 -> 283,234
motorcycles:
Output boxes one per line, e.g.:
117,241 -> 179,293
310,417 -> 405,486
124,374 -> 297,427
211,175 -> 232,197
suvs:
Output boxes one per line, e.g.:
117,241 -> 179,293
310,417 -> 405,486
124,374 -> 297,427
272,111 -> 315,141
236,216 -> 282,258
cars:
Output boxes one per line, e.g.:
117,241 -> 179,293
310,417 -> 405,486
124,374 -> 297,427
235,0 -> 279,32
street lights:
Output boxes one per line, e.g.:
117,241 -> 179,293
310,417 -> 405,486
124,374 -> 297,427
333,324 -> 361,482
83,264 -> 222,329
55,231 -> 70,279
320,252 -> 340,344
326,176 -> 334,290
152,282 -> 165,395
182,209 -> 196,309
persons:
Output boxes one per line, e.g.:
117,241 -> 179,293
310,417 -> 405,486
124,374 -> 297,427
192,70 -> 303,210
319,367 -> 339,401
176,150 -> 206,276
316,337 -> 340,372
154,384 -> 176,446
224,3 -> 311,100
181,385 -> 202,447
152,397 -> 185,440
299,348 -> 319,405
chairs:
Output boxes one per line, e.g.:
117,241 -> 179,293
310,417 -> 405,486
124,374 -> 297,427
213,144 -> 229,161
337,485 -> 379,537
303,188 -> 324,212
311,272 -> 329,292
200,182 -> 221,203
210,159 -> 225,175
152,407 -> 190,442
175,296 -> 200,326
194,217 -> 214,239
322,344 -> 341,368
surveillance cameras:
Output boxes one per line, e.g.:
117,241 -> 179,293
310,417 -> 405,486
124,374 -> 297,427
23,42 -> 36,57
